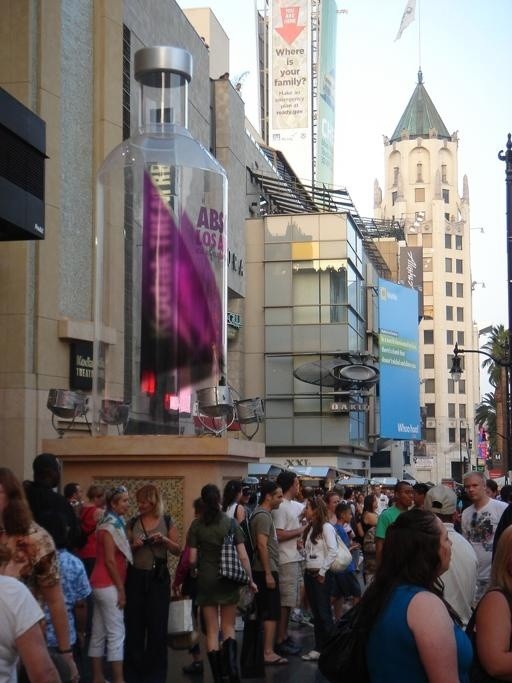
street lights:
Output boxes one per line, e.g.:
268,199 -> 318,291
447,340 -> 512,473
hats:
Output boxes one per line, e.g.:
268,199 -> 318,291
423,484 -> 457,516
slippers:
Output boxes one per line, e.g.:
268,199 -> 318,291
264,657 -> 290,666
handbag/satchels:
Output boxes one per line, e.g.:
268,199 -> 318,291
219,535 -> 251,586
324,535 -> 353,574
153,558 -> 168,570
167,585 -> 201,651
314,611 -> 370,683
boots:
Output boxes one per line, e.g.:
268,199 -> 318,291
207,649 -> 224,683
222,636 -> 240,683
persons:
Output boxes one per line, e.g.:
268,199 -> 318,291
321,472 -> 510,631
358,509 -> 474,682
181,471 -> 337,683
0,455 -> 180,683
466,526 -> 511,682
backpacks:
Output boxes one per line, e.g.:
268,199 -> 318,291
67,507 -> 96,549
239,512 -> 271,565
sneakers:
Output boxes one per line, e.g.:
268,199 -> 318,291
302,650 -> 320,661
293,609 -> 315,628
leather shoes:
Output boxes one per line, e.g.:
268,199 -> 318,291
274,638 -> 303,657
182,661 -> 205,678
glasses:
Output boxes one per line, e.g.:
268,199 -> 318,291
110,485 -> 129,501
